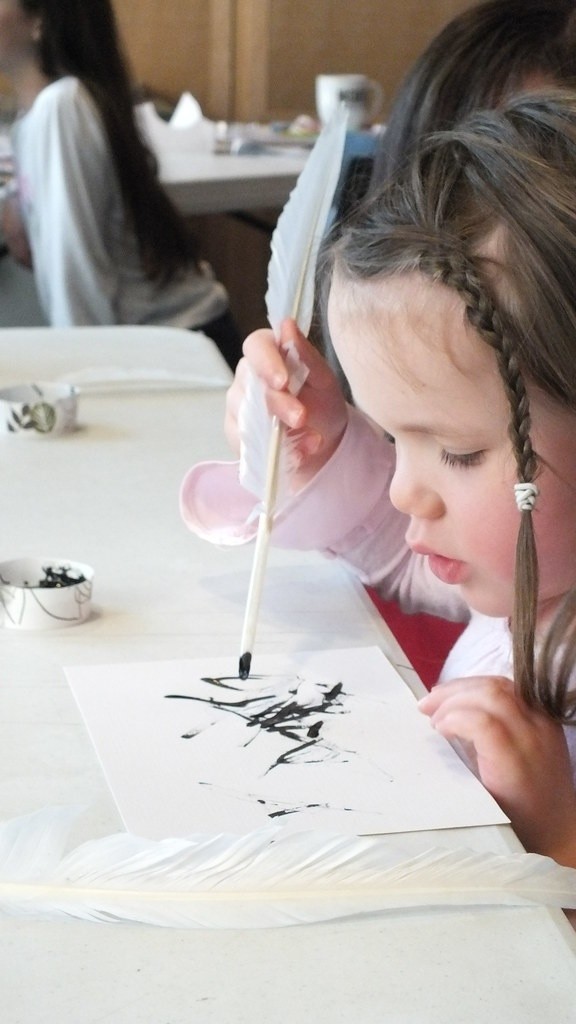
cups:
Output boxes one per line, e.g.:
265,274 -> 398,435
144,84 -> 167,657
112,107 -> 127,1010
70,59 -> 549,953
0,557 -> 95,631
0,381 -> 80,440
314,72 -> 385,136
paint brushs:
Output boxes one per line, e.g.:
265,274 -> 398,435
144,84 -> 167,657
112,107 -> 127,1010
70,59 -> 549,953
235,94 -> 349,681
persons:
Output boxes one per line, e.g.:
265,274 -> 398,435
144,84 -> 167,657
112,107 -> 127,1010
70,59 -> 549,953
360,1 -> 574,697
0,0 -> 240,371
225,85 -> 576,871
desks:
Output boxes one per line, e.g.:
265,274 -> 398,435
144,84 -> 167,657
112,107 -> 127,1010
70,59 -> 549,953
157,133 -> 318,217
0,327 -> 575,1024
